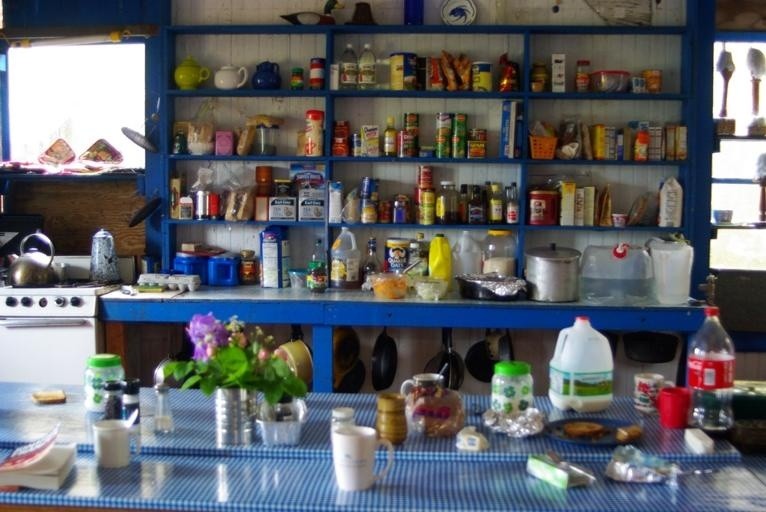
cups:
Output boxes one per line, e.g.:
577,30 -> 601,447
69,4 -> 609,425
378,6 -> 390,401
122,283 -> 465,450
92,417 -> 142,469
633,373 -> 676,413
611,214 -> 628,228
397,372 -> 444,397
331,426 -> 393,495
652,384 -> 692,431
714,209 -> 735,224
630,68 -> 664,94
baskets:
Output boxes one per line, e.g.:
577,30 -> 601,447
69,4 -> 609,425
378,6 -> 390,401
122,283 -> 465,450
529,135 -> 558,160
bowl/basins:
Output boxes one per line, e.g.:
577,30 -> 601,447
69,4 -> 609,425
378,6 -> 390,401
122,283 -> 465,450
413,278 -> 448,300
590,71 -> 630,92
371,274 -> 410,300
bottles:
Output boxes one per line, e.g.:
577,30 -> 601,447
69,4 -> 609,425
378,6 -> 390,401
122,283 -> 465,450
338,44 -> 374,89
171,129 -> 186,155
330,406 -> 355,427
310,239 -> 328,294
256,125 -> 279,155
381,117 -> 397,157
687,306 -> 738,440
289,66 -> 304,90
360,236 -> 382,280
379,199 -> 411,224
102,378 -> 143,426
407,233 -> 427,277
534,62 -> 548,91
575,57 -> 591,93
150,380 -> 177,435
435,180 -> 457,225
457,180 -> 519,224
60,262 -> 70,279
375,393 -> 408,444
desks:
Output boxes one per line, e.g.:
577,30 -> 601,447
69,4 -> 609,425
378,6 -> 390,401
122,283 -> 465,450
1,382 -> 765,512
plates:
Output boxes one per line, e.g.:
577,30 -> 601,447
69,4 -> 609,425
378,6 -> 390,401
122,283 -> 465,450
543,417 -> 631,447
440,0 -> 479,26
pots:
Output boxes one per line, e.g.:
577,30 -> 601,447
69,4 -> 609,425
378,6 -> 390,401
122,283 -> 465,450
524,242 -> 582,304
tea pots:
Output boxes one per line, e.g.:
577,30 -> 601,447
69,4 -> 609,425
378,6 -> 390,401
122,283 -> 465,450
87,227 -> 119,284
175,53 -> 282,91
6,230 -> 56,286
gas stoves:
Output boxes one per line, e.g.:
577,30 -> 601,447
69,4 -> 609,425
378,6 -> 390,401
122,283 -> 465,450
0,277 -> 115,316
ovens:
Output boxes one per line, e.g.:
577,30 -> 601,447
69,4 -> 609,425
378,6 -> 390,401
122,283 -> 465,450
0,315 -> 103,388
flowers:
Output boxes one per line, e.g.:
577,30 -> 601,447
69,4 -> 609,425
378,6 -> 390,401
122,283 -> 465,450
163,312 -> 307,405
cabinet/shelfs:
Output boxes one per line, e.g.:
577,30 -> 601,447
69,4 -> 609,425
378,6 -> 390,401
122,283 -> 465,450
161,26 -> 691,393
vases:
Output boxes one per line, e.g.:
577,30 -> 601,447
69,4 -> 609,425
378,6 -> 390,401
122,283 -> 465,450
215,387 -> 258,445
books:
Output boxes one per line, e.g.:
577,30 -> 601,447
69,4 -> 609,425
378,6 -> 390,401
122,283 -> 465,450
0,421 -> 79,491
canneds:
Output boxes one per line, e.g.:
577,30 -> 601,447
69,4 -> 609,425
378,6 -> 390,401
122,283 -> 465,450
307,261 -> 328,291
272,180 -> 293,197
359,176 -> 393,224
397,112 -> 487,159
388,52 -> 419,90
470,61 -> 493,92
529,190 -> 560,225
480,229 -> 517,278
